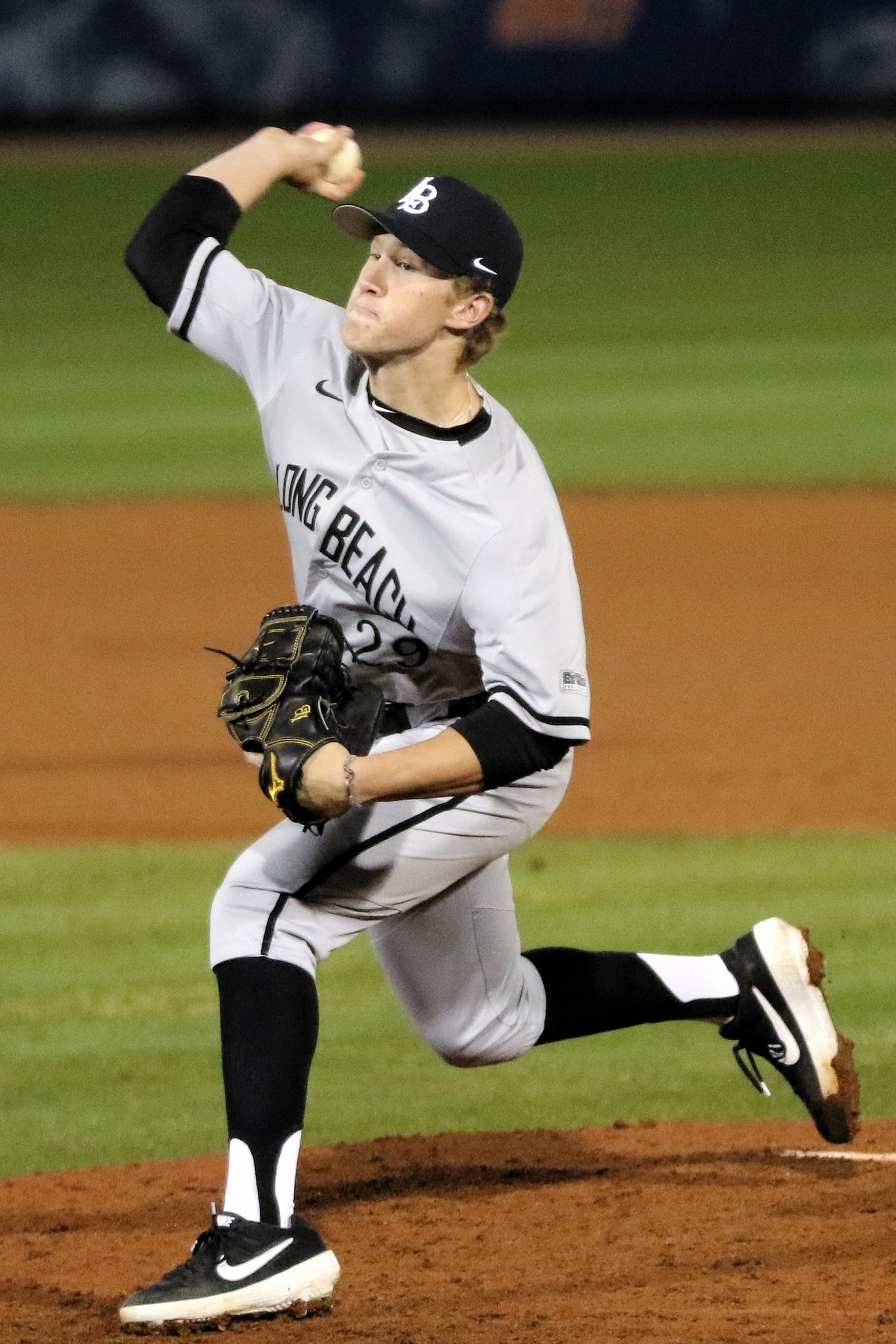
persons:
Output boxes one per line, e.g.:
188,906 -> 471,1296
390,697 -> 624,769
122,125 -> 861,1338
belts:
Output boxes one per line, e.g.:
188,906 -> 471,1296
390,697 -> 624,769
382,690 -> 488,734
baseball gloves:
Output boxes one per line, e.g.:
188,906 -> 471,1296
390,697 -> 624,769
202,603 -> 389,837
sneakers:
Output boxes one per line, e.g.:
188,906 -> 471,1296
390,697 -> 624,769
719,917 -> 860,1144
119,1202 -> 342,1335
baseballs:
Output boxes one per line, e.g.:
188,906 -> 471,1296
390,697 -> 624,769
305,129 -> 363,184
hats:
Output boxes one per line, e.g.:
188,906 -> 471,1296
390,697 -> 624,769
331,175 -> 524,309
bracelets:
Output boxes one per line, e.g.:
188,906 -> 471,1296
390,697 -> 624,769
342,754 -> 362,814
